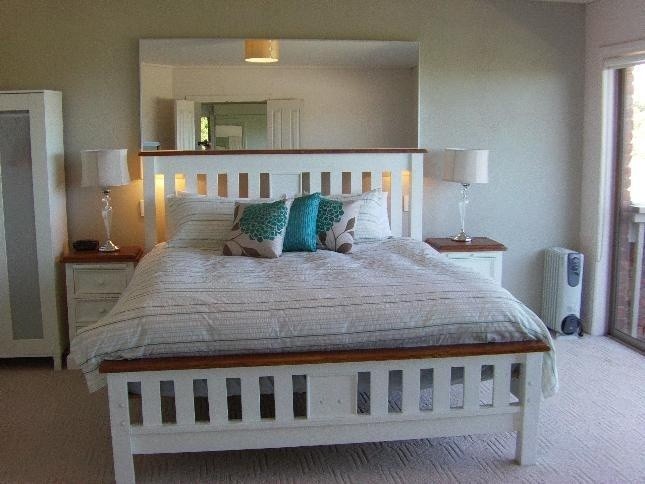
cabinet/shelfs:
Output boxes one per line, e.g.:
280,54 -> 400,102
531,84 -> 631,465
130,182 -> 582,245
0,88 -> 71,372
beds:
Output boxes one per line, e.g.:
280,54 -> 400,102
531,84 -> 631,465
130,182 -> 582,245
97,148 -> 554,483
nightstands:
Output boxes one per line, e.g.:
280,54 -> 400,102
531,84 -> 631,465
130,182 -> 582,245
427,236 -> 508,297
60,244 -> 143,345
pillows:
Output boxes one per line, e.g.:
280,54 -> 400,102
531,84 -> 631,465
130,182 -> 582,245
162,183 -> 392,260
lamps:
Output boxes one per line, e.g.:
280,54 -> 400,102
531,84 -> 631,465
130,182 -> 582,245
442,147 -> 490,243
245,38 -> 280,63
79,146 -> 132,254
